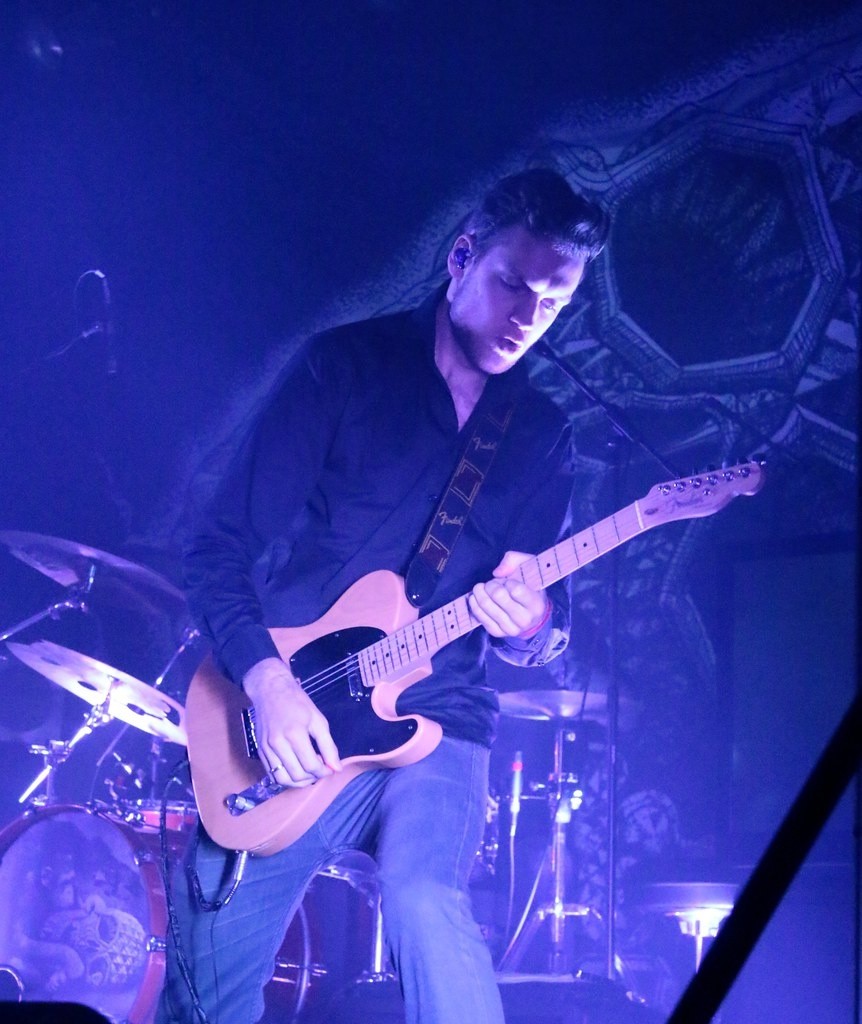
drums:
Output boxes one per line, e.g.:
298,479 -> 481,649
135,804 -> 199,861
256,848 -> 384,1023
0,801 -> 327,1024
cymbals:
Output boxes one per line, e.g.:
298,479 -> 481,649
4,637 -> 187,745
500,690 -> 642,721
0,529 -> 186,618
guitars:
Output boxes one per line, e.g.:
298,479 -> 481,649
186,452 -> 766,859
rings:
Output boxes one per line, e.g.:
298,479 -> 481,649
271,763 -> 285,774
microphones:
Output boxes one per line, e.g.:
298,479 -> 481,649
222,851 -> 248,907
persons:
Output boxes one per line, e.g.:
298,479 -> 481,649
148,164 -> 614,1023
